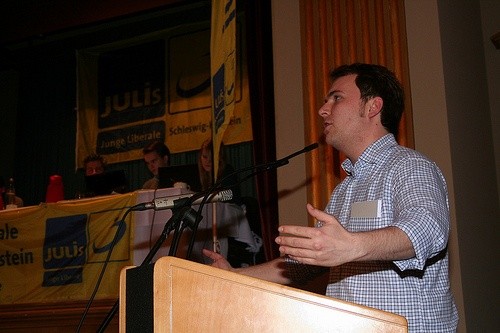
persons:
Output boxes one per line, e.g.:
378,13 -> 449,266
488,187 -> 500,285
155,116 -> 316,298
84,154 -> 105,176
142,141 -> 172,189
188,138 -> 241,205
203,62 -> 459,333
0,176 -> 24,210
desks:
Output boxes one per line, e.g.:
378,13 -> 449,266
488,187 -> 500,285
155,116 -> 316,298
0,188 -> 253,305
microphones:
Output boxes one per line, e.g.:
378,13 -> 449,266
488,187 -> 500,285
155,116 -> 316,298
131,143 -> 319,259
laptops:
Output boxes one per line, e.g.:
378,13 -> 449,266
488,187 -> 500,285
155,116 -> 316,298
157,163 -> 202,192
85,168 -> 133,198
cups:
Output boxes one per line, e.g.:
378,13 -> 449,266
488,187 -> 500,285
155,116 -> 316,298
174,181 -> 191,190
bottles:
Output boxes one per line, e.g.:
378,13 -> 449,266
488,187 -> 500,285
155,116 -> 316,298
5,178 -> 17,209
